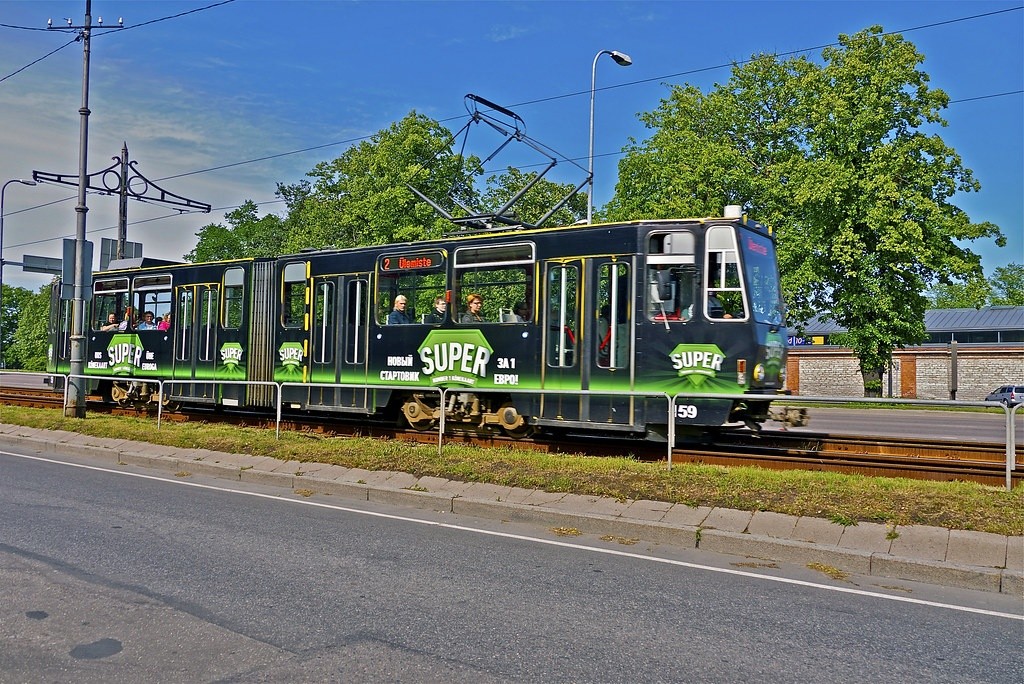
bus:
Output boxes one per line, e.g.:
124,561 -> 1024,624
44,214 -> 809,444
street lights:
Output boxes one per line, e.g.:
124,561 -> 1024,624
559,219 -> 588,367
588,49 -> 632,225
0,179 -> 36,314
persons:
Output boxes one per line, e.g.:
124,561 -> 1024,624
506,301 -> 529,323
158,312 -> 171,331
424,297 -> 447,323
599,262 -> 629,370
135,311 -> 158,330
708,290 -> 732,319
462,294 -> 488,323
388,294 -> 411,324
155,316 -> 163,325
99,311 -> 130,332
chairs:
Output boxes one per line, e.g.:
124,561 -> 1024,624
52,307 -> 626,370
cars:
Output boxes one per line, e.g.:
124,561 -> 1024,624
984,385 -> 1024,408
